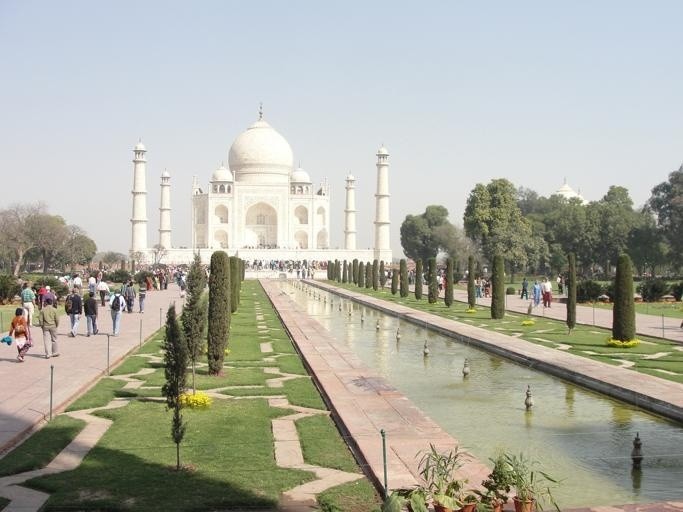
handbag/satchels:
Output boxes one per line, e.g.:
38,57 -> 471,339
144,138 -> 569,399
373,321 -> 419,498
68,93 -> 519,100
15,324 -> 25,334
66,296 -> 73,312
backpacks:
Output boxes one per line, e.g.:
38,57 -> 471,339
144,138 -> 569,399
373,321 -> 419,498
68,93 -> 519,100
112,294 -> 121,311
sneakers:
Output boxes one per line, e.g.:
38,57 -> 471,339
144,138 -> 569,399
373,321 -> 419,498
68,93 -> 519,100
71,331 -> 75,337
46,355 -> 51,358
53,353 -> 59,357
94,329 -> 98,334
17,356 -> 25,362
